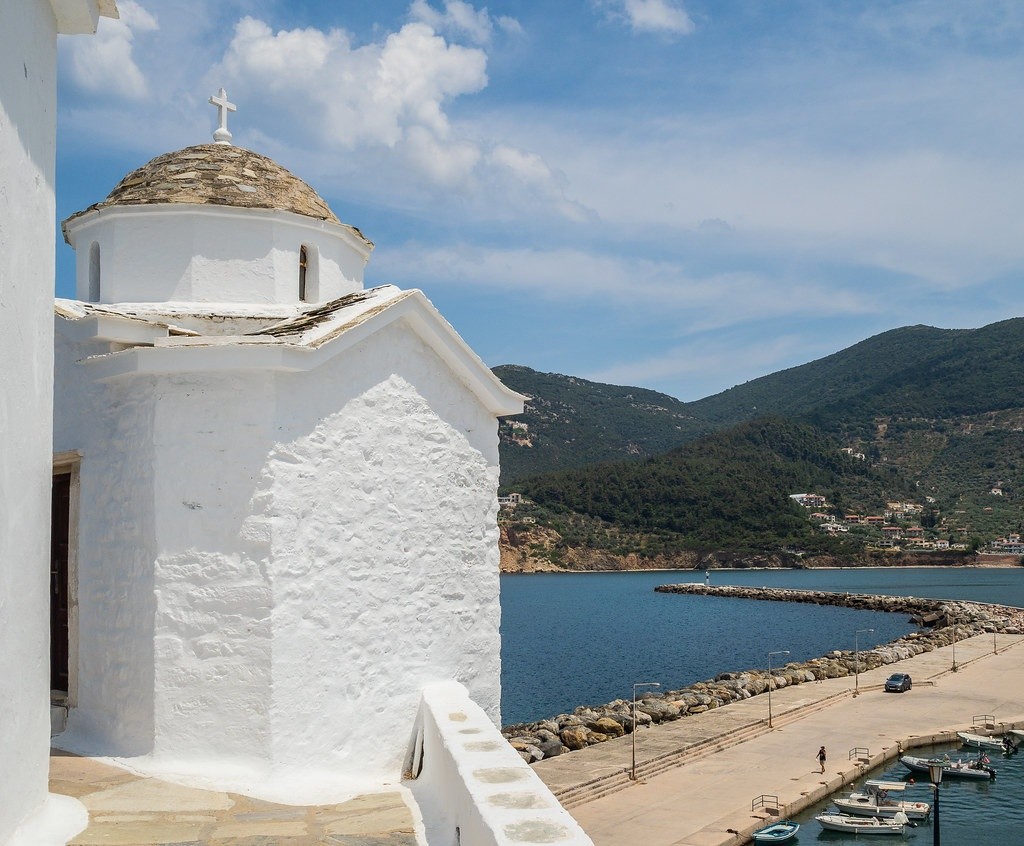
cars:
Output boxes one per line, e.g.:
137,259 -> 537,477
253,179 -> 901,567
885,673 -> 912,693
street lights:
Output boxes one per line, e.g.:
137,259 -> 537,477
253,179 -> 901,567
855,629 -> 874,694
768,651 -> 790,728
993,610 -> 1013,653
633,683 -> 660,779
926,758 -> 951,846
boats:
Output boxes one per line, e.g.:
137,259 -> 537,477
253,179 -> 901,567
751,820 -> 800,846
815,811 -> 917,834
957,733 -> 1018,752
865,780 -> 936,796
830,793 -> 932,820
901,756 -> 998,782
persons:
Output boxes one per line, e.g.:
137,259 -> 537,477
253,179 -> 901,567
815,746 -> 826,775
